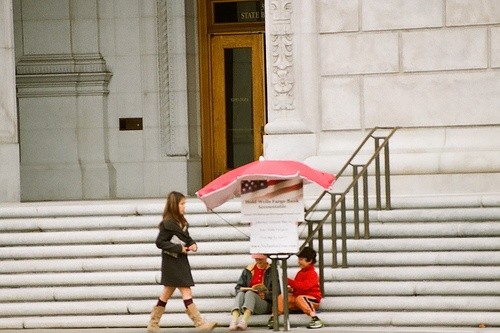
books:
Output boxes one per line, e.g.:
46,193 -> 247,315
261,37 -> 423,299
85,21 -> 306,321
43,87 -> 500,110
240,283 -> 268,294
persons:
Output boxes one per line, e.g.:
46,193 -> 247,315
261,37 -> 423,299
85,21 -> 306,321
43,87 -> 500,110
147,191 -> 218,333
268,247 -> 325,328
228,254 -> 281,331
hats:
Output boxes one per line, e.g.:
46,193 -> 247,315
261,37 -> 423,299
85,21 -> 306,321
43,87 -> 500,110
251,254 -> 267,258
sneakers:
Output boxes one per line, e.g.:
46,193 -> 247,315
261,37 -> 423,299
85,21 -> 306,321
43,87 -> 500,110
268,316 -> 274,326
306,318 -> 324,328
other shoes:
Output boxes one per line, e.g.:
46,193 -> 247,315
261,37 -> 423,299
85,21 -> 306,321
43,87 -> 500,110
228,320 -> 238,330
237,320 -> 247,330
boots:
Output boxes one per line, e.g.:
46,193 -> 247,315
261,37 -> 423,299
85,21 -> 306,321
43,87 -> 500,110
147,306 -> 165,333
185,303 -> 217,333
